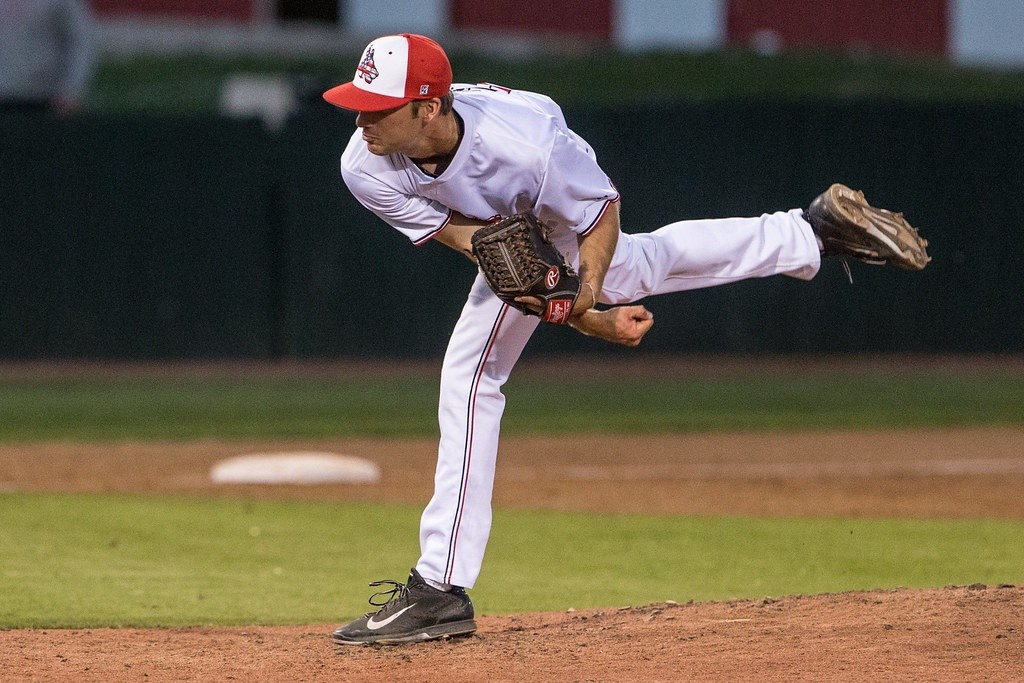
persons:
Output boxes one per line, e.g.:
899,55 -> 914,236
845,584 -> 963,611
323,33 -> 931,645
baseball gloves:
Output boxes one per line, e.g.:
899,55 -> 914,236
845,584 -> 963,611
470,210 -> 582,326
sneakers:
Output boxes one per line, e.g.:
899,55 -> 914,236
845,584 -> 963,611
331,568 -> 478,646
809,182 -> 932,271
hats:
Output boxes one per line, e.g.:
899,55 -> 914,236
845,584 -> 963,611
323,33 -> 453,111
